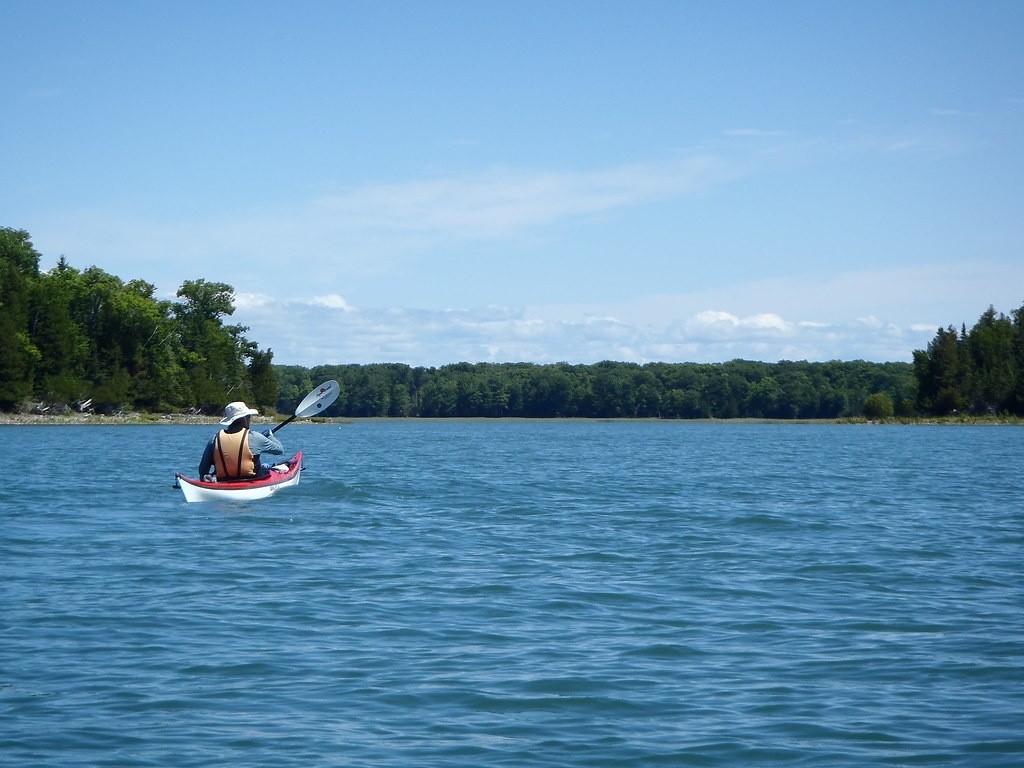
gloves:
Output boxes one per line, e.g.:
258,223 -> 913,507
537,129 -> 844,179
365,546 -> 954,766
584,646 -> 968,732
261,429 -> 274,439
199,473 -> 213,482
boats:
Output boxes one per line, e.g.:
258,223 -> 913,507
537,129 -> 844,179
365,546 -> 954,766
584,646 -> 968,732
175,451 -> 303,503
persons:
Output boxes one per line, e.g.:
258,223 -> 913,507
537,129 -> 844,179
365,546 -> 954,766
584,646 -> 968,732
199,402 -> 284,483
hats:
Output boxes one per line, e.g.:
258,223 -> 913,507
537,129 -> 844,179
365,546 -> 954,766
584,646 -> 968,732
219,401 -> 258,426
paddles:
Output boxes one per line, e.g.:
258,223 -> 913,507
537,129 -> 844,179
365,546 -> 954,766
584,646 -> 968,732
209,378 -> 341,478
